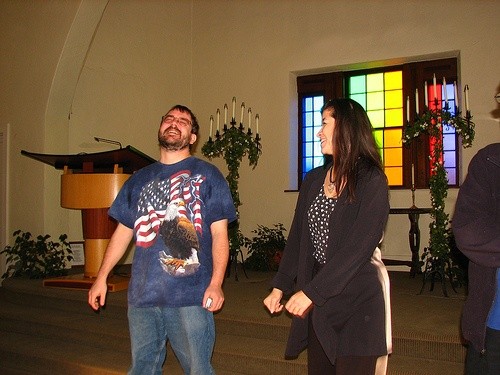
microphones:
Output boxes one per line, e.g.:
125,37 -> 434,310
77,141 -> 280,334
95,136 -> 122,149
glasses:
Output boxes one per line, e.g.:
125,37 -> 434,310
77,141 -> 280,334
162,115 -> 193,127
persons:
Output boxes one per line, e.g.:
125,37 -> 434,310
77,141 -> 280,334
264,98 -> 392,375
452,142 -> 500,375
88,105 -> 238,375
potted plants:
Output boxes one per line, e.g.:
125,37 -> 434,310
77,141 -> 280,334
242,222 -> 287,272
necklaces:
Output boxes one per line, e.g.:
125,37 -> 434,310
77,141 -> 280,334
327,168 -> 336,194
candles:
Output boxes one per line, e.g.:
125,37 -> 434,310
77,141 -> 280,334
443,76 -> 448,102
415,88 -> 419,113
407,95 -> 410,123
433,73 -> 437,98
424,80 -> 428,106
464,85 -> 469,109
241,103 -> 246,123
454,81 -> 458,108
232,97 -> 235,119
248,107 -> 251,129
412,163 -> 414,185
255,114 -> 258,134
224,104 -> 228,125
209,115 -> 214,136
217,109 -> 220,131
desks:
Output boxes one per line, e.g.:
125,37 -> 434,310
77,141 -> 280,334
381,207 -> 432,277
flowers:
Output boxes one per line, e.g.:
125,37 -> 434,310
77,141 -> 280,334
202,130 -> 261,248
401,110 -> 476,282
